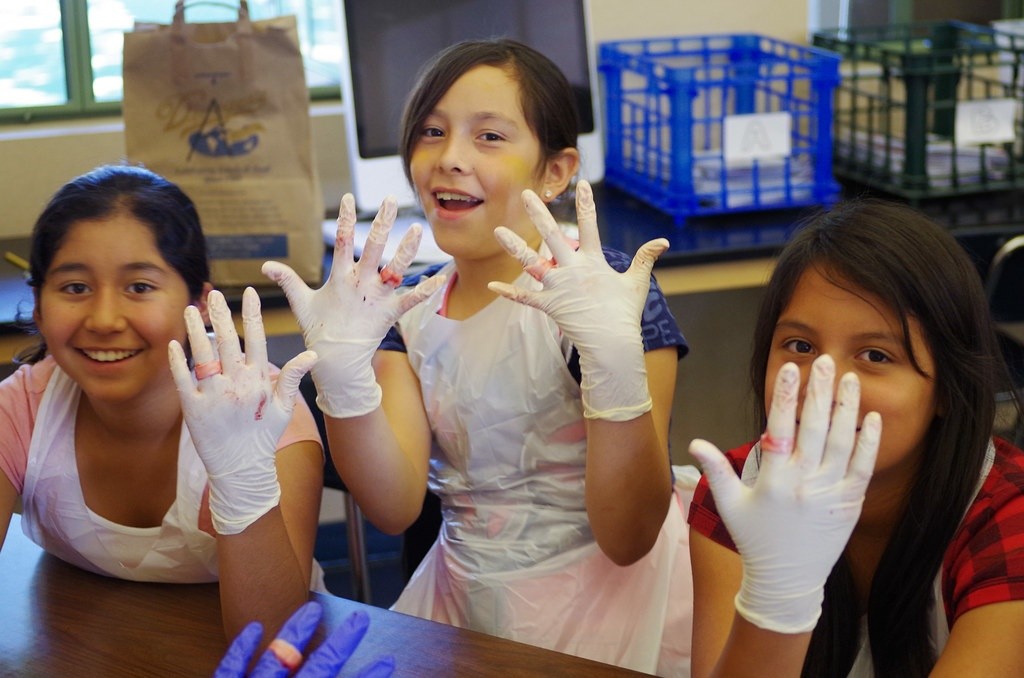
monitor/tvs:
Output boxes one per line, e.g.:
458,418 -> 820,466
334,0 -> 604,216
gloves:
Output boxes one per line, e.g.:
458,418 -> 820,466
169,285 -> 316,535
487,179 -> 669,421
262,194 -> 447,417
689,354 -> 881,634
217,602 -> 394,678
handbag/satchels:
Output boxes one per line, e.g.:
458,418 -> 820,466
123,1 -> 321,299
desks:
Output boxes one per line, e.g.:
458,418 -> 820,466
0,255 -> 778,367
0,511 -> 660,677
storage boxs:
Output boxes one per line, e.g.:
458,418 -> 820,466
812,20 -> 1023,202
593,31 -> 841,224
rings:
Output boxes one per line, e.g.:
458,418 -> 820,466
195,361 -> 220,380
757,434 -> 795,453
381,264 -> 403,288
267,637 -> 305,671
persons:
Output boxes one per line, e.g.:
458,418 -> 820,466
2,165 -> 324,664
212,600 -> 397,678
261,40 -> 693,678
685,196 -> 1024,678
